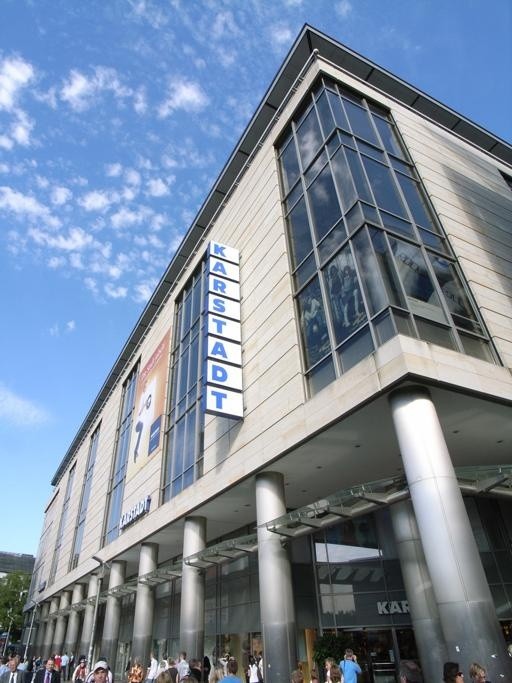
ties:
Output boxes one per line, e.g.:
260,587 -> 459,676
45,672 -> 51,683
10,673 -> 15,683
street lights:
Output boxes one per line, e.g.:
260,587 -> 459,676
85,557 -> 111,675
2,614 -> 15,656
23,600 -> 41,661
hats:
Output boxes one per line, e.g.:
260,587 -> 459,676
93,659 -> 110,672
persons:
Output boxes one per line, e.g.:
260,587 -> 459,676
328,265 -> 343,324
291,649 -> 363,683
399,660 -> 423,683
134,394 -> 152,463
469,662 -> 492,683
342,266 -> 362,328
443,662 -> 464,683
300,295 -> 323,351
0,650 -> 264,683
